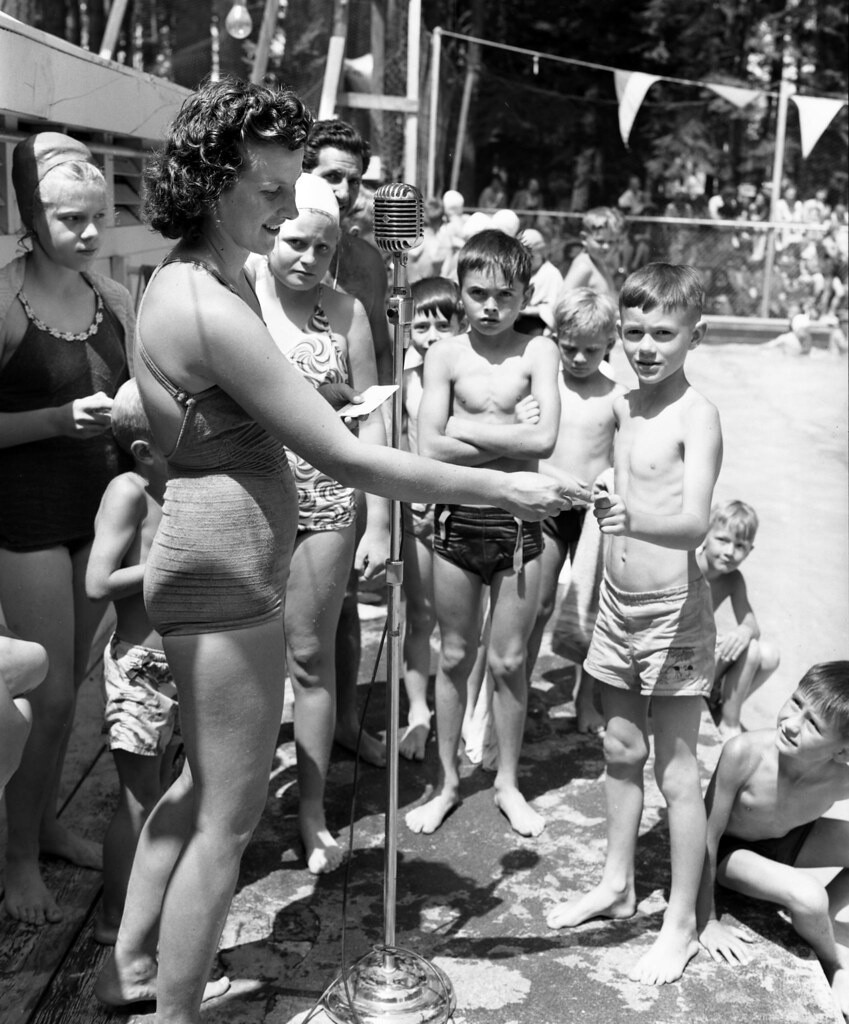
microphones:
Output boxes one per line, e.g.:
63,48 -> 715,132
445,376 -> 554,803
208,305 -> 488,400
374,184 -> 424,253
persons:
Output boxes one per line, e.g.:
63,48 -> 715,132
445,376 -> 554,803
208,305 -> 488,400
0,131 -> 241,950
257,144 -> 849,1017
92,76 -> 602,1024
